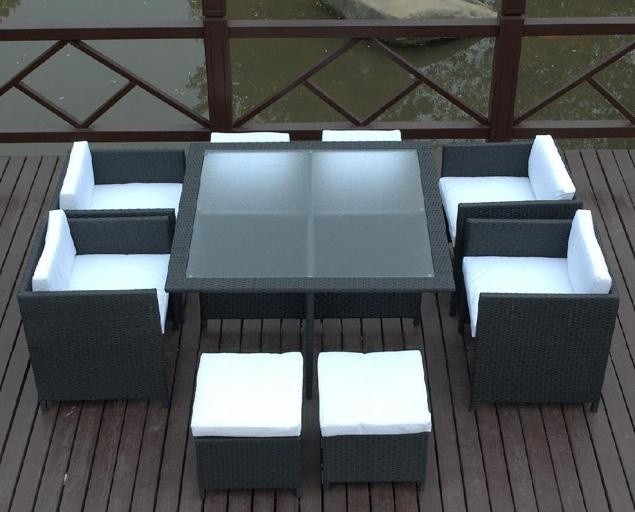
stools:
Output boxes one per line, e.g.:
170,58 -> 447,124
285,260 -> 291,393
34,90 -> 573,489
186,347 -> 433,500
208,126 -> 403,144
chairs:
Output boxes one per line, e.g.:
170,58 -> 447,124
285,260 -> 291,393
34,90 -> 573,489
16,210 -> 183,402
56,134 -> 186,234
436,131 -> 583,267
450,209 -> 620,413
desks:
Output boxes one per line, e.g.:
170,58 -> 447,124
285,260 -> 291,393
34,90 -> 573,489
164,140 -> 458,402
